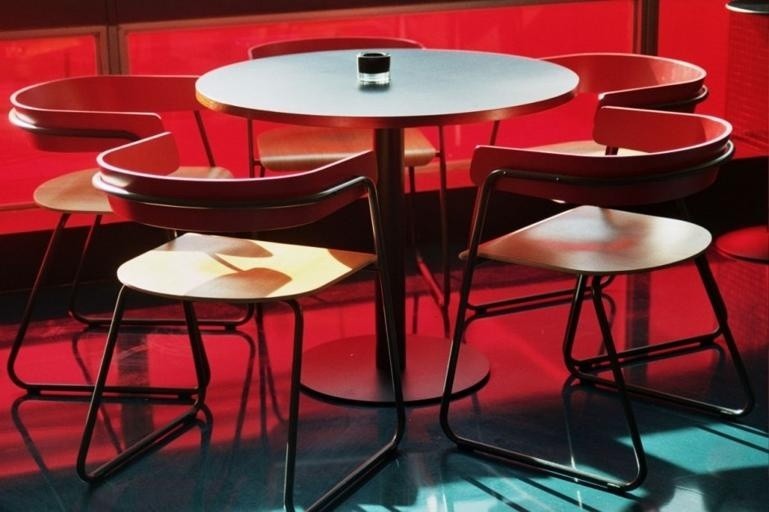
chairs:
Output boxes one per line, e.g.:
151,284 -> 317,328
77,131 -> 405,510
462,51 -> 728,369
247,36 -> 450,310
7,75 -> 254,394
437,103 -> 755,493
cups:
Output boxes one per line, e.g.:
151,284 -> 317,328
357,51 -> 391,93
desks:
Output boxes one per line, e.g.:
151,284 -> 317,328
196,49 -> 580,410
